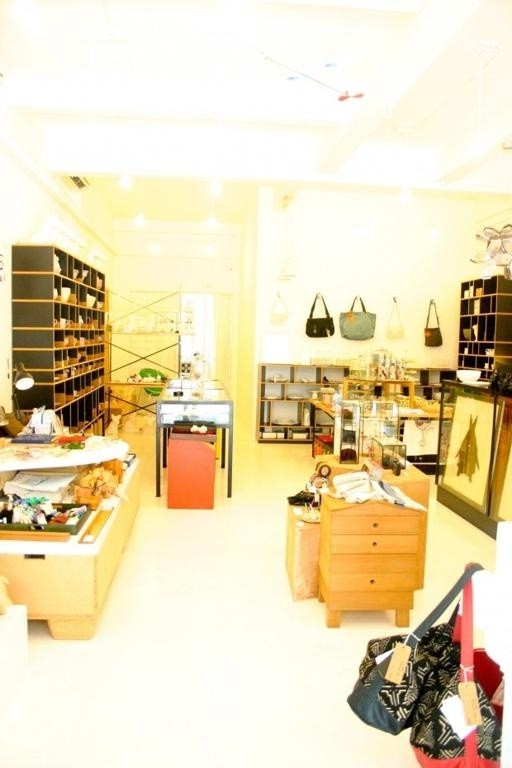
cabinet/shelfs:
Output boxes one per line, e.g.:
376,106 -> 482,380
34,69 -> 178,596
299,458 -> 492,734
256,363 -> 348,444
318,485 -> 417,628
0,435 -> 143,641
12,245 -> 106,436
438,380 -> 512,539
456,276 -> 511,375
343,367 -> 456,473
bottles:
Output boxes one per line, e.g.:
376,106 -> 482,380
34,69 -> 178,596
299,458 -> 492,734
392,459 -> 400,476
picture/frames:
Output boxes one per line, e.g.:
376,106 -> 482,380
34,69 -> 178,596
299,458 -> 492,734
440,391 -> 503,516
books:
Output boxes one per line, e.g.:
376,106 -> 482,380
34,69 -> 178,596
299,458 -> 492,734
2,471 -> 77,503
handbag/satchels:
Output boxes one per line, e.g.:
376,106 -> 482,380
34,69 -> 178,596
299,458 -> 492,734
424,299 -> 442,347
305,292 -> 335,338
490,365 -> 512,397
339,296 -> 377,340
384,300 -> 405,339
347,561 -> 505,768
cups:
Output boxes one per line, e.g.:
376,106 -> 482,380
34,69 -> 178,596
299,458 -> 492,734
62,287 -> 71,302
54,318 -> 73,328
368,363 -> 377,378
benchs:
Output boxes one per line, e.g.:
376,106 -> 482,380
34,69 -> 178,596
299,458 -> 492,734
362,457 -> 430,514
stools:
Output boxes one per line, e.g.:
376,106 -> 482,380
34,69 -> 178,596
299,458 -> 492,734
286,493 -> 320,601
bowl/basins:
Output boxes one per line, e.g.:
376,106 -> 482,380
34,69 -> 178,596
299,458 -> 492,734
462,328 -> 471,340
456,370 -> 482,383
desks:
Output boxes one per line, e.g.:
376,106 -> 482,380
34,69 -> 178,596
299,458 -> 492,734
155,376 -> 233,498
